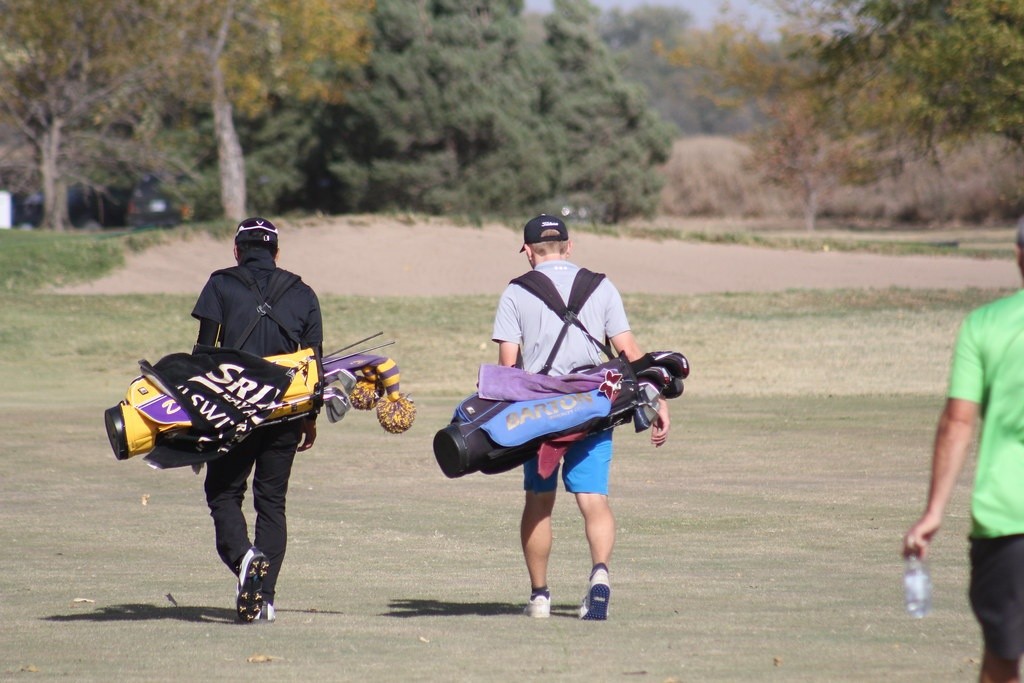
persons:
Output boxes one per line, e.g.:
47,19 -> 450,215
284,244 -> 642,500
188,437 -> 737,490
492,212 -> 670,619
190,218 -> 323,623
901,214 -> 1024,683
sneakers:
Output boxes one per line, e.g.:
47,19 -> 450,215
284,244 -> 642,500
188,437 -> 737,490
522,595 -> 551,617
236,546 -> 270,622
578,569 -> 610,621
253,598 -> 276,623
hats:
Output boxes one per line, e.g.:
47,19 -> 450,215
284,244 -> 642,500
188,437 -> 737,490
234,218 -> 278,245
519,214 -> 569,253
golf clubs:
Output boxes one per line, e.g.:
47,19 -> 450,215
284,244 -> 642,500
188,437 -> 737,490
639,381 -> 661,423
321,368 -> 358,424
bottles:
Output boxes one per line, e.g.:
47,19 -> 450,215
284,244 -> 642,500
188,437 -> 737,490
903,553 -> 934,619
